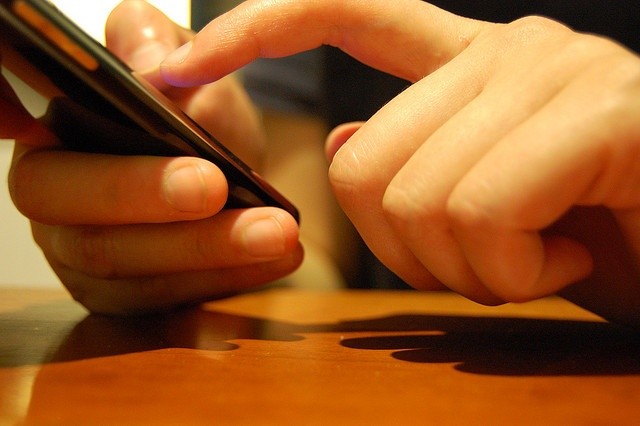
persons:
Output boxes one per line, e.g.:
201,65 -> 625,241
1,0 -> 640,326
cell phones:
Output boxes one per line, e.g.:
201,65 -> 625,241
0,0 -> 300,225
